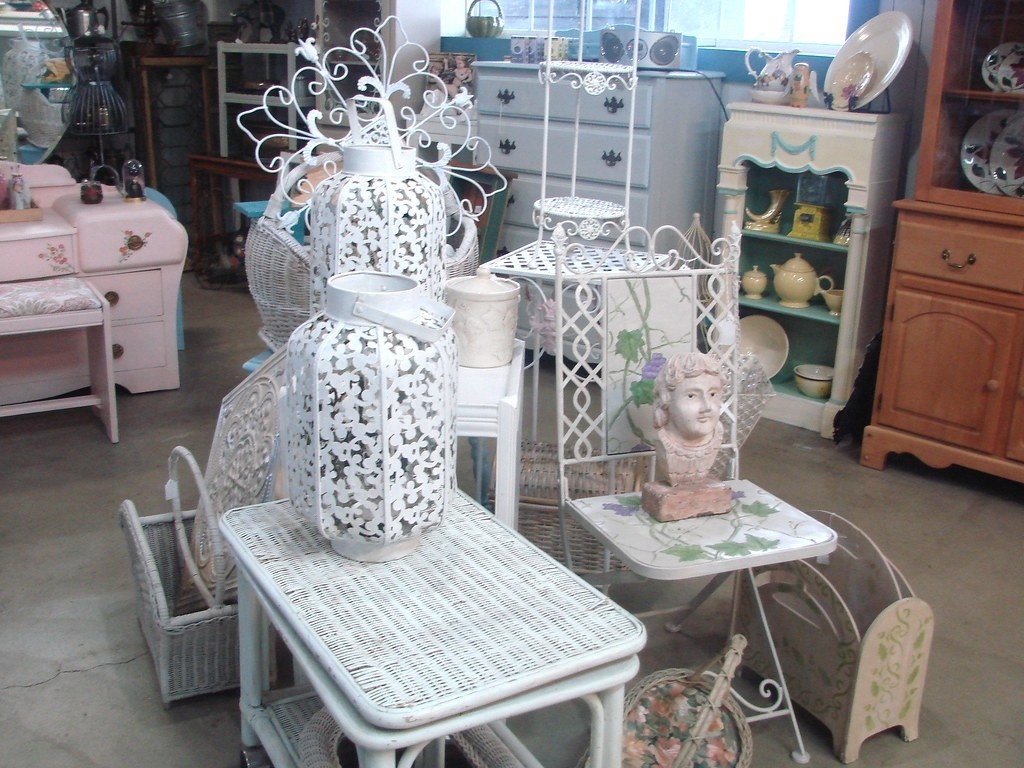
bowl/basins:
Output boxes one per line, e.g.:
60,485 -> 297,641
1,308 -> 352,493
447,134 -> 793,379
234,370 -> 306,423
793,363 -> 834,399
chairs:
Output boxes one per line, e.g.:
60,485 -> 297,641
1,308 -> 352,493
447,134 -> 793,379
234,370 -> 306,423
556,223 -> 837,768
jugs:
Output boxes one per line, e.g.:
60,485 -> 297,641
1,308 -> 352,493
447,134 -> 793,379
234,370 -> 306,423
745,47 -> 800,103
769,252 -> 834,309
741,265 -> 768,299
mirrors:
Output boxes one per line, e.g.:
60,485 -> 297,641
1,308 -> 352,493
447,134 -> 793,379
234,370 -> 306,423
0,0 -> 78,166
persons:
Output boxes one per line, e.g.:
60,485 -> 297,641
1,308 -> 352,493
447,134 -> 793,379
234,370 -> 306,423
653,353 -> 725,486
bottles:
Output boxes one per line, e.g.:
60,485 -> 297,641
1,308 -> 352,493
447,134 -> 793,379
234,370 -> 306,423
8,172 -> 31,210
445,267 -> 521,369
789,62 -> 809,108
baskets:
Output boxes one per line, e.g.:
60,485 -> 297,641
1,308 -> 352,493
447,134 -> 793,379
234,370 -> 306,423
118,446 -> 241,703
465,0 -> 505,37
245,150 -> 479,352
21,62 -> 77,148
299,705 -> 543,768
488,438 -> 648,573
576,634 -> 753,768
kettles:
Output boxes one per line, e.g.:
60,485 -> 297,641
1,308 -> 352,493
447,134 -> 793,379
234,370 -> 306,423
60,0 -> 109,38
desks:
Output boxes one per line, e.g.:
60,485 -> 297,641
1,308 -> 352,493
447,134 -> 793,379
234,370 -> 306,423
187,151 -> 274,261
212,492 -> 647,768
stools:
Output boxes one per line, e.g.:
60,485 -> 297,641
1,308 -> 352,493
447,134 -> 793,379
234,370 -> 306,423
0,277 -> 118,446
456,334 -> 525,529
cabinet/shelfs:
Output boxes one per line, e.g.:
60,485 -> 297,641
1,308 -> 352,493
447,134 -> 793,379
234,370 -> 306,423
221,43 -> 314,160
314,0 -> 444,155
858,0 -> 1024,485
461,67 -> 721,367
142,52 -> 225,250
706,102 -> 906,443
0,162 -> 189,407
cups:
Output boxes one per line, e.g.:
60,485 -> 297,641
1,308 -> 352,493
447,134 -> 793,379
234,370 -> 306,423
824,289 -> 843,316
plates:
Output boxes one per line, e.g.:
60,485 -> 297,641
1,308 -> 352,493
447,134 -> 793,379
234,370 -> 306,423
823,10 -> 914,109
960,40 -> 1024,200
740,314 -> 790,379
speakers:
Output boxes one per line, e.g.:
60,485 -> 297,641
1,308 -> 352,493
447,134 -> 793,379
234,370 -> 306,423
598,29 -> 698,72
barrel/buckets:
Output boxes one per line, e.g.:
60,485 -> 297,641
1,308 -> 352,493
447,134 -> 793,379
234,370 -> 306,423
155,0 -> 208,49
